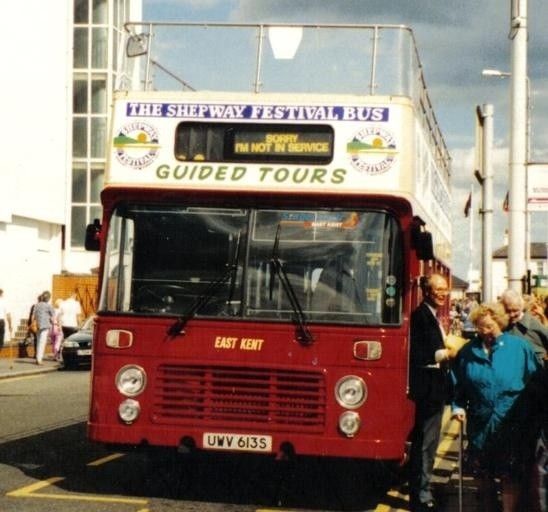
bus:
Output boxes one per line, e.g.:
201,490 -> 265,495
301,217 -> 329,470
83,20 -> 454,485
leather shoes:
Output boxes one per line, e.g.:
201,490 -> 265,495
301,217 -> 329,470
411,501 -> 443,511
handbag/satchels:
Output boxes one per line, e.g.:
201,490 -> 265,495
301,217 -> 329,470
29,318 -> 37,333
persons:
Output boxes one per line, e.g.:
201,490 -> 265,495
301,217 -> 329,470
27,289 -> 56,366
0,289 -> 13,352
309,226 -> 370,326
49,297 -> 66,362
443,286 -> 548,512
55,287 -> 85,365
407,272 -> 459,511
27,292 -> 43,363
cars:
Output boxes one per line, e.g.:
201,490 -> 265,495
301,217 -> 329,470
61,314 -> 95,370
460,313 -> 479,340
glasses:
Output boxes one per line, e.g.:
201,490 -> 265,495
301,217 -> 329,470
474,322 -> 497,334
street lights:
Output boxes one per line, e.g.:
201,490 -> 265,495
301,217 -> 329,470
481,67 -> 532,294
